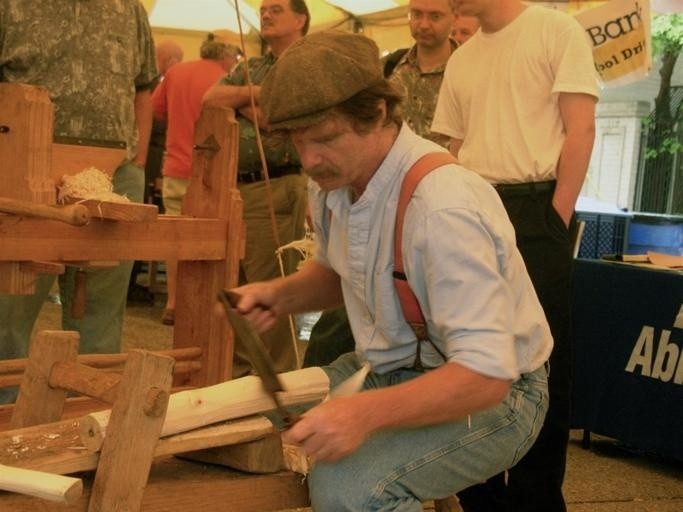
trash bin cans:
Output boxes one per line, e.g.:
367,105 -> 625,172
626,213 -> 683,255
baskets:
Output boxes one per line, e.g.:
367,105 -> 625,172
574,212 -> 625,259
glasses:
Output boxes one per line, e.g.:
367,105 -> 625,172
409,9 -> 444,22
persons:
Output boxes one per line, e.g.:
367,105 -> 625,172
212,28 -> 556,509
0,0 -> 159,411
384,0 -> 463,146
446,14 -> 479,48
146,28 -> 241,326
122,40 -> 184,308
427,0 -> 602,511
197,0 -> 316,379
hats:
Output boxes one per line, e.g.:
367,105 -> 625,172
209,28 -> 244,53
258,32 -> 382,133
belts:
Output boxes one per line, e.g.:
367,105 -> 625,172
238,164 -> 302,184
494,182 -> 554,196
